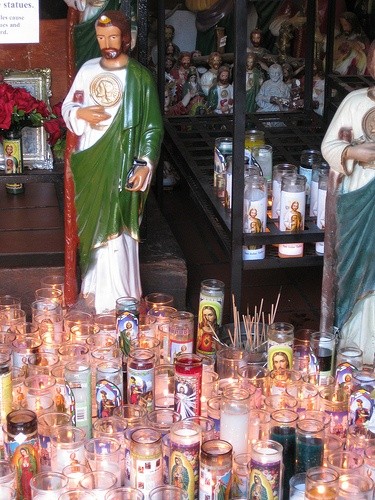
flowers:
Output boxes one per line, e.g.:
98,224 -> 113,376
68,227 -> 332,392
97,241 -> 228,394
0,82 -> 58,134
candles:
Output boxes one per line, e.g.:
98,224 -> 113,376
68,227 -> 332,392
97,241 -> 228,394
0,292 -> 375,500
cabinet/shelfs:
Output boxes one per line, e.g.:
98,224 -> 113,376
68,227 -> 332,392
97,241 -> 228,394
158,0 -> 336,323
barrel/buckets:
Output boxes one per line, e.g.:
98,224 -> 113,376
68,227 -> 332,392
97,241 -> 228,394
210,322 -> 269,378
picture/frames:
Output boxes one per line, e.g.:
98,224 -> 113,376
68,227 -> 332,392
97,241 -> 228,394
0,68 -> 53,170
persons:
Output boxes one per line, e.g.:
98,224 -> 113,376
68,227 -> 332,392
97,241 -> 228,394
146,12 -> 368,115
61,11 -> 164,314
320,41 -> 375,365
64,0 -> 138,77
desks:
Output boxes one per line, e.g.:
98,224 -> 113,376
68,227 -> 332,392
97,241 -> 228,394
0,184 -> 66,256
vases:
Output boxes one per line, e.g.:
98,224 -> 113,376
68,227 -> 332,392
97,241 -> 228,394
2,128 -> 23,175
